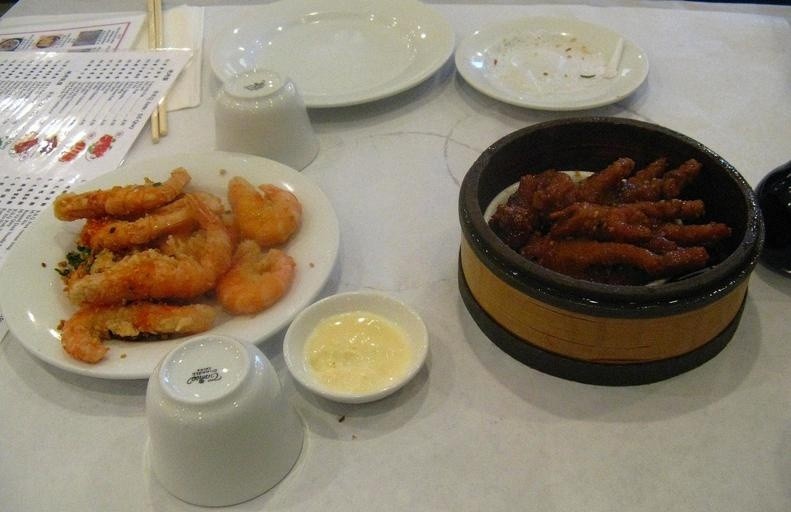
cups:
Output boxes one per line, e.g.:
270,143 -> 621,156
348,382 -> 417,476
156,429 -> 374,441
212,71 -> 319,170
144,335 -> 306,508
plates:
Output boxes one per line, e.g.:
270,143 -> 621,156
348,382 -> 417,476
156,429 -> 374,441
208,1 -> 456,108
283,291 -> 431,403
456,16 -> 651,111
756,160 -> 791,278
1,152 -> 340,379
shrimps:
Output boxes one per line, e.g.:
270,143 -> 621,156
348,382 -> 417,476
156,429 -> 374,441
52,166 -> 234,305
227,173 -> 303,247
217,238 -> 298,315
61,300 -> 223,365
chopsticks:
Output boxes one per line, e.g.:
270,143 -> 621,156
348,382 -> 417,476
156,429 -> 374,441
147,1 -> 167,142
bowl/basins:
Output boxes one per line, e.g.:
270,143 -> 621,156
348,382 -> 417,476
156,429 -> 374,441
456,113 -> 767,388
